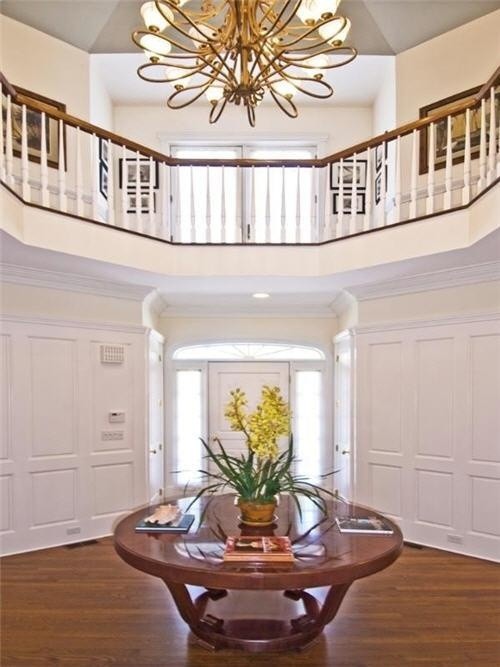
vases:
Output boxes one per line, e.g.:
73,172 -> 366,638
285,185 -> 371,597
233,495 -> 280,528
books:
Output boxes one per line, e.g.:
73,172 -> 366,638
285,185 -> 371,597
223,535 -> 295,562
334,515 -> 394,535
134,514 -> 196,533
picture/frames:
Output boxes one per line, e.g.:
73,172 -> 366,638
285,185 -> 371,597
417,80 -> 500,176
118,157 -> 159,213
3,80 -> 68,171
330,131 -> 388,214
98,137 -> 108,198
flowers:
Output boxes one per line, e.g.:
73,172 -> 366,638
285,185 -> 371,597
185,385 -> 341,527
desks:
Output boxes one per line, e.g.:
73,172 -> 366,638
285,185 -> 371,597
112,493 -> 404,656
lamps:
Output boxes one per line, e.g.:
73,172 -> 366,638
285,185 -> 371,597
133,1 -> 360,127
